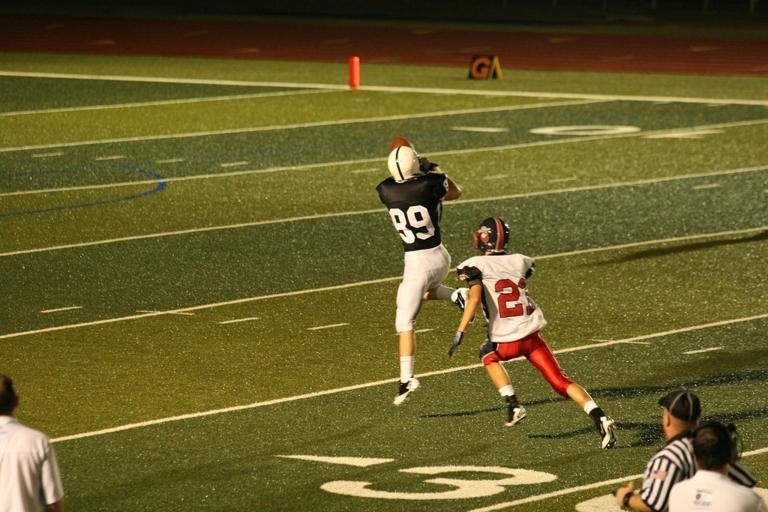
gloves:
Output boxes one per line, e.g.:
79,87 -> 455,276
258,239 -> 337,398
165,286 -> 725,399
448,331 -> 463,356
420,157 -> 437,173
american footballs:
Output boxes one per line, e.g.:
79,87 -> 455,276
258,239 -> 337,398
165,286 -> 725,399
391,137 -> 413,151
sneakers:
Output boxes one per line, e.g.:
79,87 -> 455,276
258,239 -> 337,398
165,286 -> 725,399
450,287 -> 475,321
392,376 -> 419,405
599,415 -> 618,450
505,406 -> 526,428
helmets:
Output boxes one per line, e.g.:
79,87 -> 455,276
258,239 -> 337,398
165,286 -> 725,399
473,218 -> 510,254
388,146 -> 420,183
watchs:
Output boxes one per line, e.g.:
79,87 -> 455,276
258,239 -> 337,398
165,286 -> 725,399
623,491 -> 634,509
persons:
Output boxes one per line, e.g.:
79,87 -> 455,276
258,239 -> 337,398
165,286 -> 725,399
375,137 -> 475,405
0,371 -> 64,512
449,217 -> 616,450
669,422 -> 768,512
615,390 -> 758,512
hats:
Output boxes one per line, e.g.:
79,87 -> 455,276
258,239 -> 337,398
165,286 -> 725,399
658,391 -> 700,421
